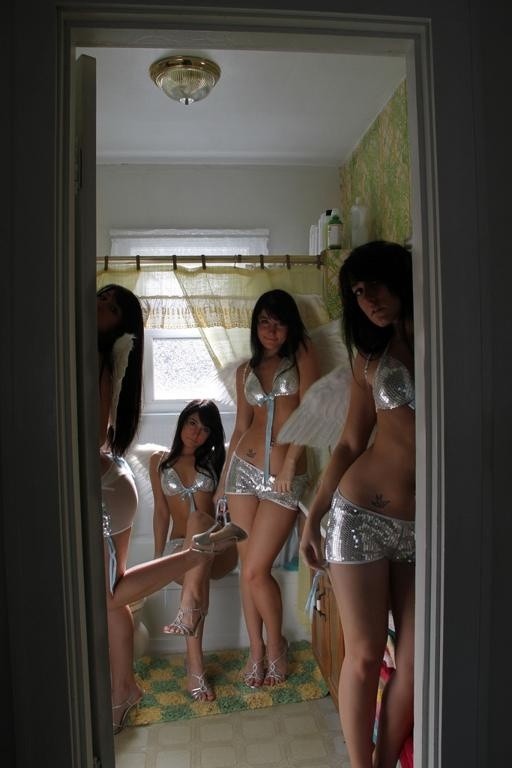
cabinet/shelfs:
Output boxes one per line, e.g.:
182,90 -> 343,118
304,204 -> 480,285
303,548 -> 355,709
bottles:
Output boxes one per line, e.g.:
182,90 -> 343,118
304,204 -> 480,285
309,197 -> 370,256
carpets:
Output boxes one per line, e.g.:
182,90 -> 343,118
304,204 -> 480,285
123,639 -> 331,727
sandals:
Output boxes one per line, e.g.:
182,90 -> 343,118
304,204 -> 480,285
244,641 -> 265,688
264,637 -> 288,685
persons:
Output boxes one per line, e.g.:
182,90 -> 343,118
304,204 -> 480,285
148,399 -> 238,704
94,282 -> 249,736
297,237 -> 418,768
211,288 -> 321,690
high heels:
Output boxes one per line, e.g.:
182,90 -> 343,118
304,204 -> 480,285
191,521 -> 248,553
163,605 -> 208,638
111,683 -> 145,735
191,671 -> 214,702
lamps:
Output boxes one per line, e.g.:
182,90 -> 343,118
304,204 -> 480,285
150,54 -> 222,107
